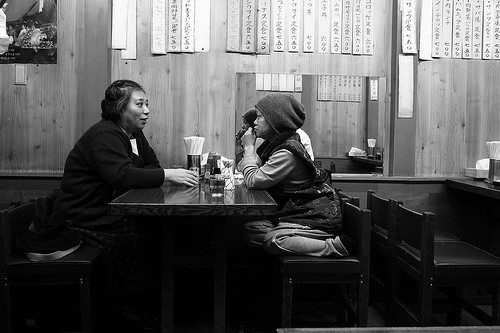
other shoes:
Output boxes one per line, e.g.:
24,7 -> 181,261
340,233 -> 359,257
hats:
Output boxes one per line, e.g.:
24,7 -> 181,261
242,108 -> 257,128
254,94 -> 306,134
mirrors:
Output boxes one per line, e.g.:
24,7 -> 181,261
235,72 -> 386,177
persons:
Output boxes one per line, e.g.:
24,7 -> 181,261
235,107 -> 315,172
51,80 -> 199,322
235,94 -> 350,258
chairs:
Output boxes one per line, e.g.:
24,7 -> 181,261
278,197 -> 372,328
37,191 -> 58,224
388,200 -> 500,327
334,188 -> 360,207
367,189 -> 461,327
0,201 -> 103,333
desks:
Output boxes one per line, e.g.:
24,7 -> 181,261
346,152 -> 382,172
445,178 -> 500,200
107,173 -> 278,333
